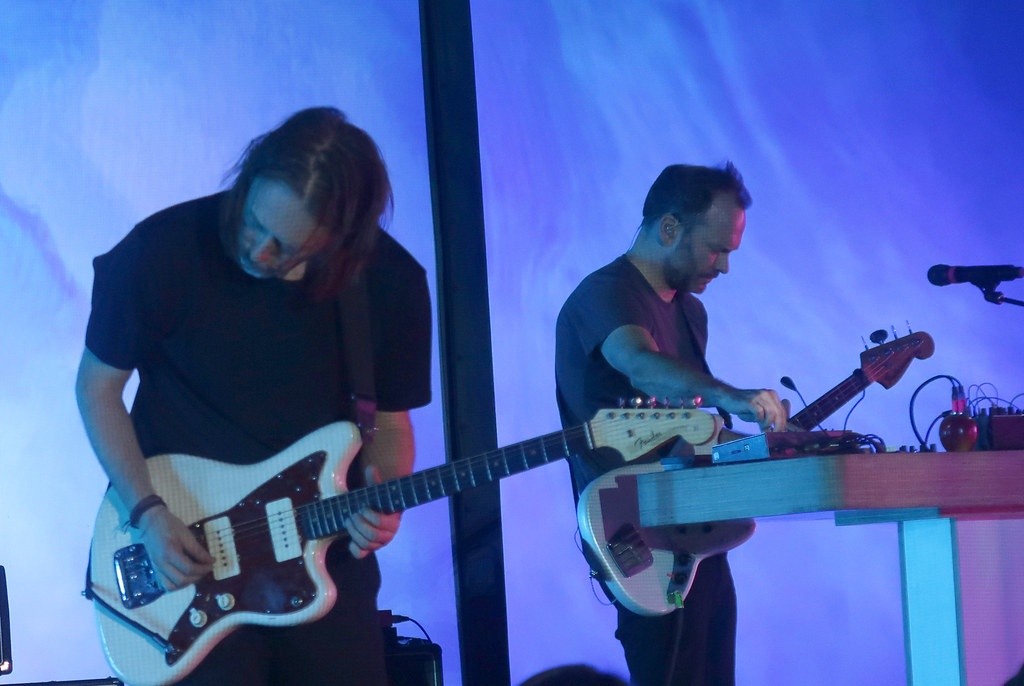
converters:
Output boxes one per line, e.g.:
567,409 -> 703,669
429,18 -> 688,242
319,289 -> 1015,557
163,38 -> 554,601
379,610 -> 397,640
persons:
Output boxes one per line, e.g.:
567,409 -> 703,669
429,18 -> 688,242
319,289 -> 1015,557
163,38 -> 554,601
76,107 -> 432,685
555,163 -> 793,686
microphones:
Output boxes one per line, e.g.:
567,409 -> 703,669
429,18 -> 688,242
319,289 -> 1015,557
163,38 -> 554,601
928,264 -> 1024,287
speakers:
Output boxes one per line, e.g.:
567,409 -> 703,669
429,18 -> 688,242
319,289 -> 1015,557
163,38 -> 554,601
381,639 -> 444,686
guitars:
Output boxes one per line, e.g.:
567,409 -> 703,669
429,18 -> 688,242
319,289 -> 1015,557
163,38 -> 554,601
574,317 -> 936,617
90,395 -> 727,686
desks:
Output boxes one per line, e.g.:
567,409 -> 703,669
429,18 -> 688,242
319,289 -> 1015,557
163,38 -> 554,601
639,449 -> 1024,686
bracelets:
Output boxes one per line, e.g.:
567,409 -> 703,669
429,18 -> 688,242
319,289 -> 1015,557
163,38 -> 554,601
123,495 -> 167,529
